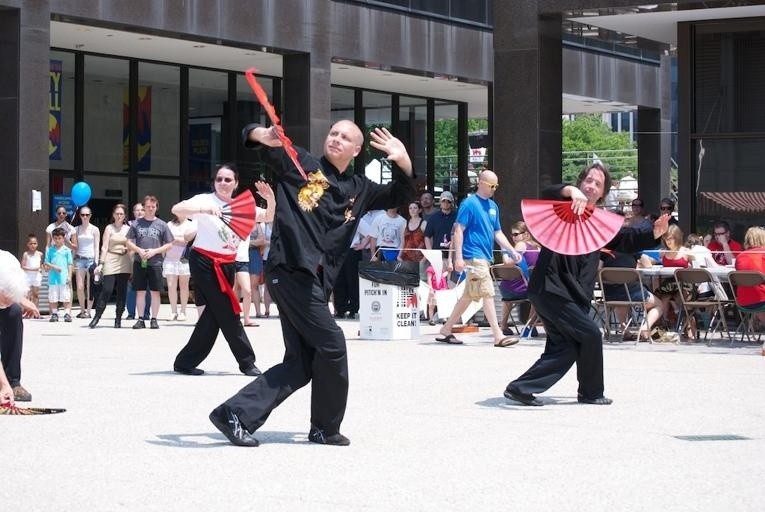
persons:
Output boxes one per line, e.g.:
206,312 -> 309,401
19,234 -> 44,318
171,165 -> 277,379
0,248 -> 40,409
209,115 -> 414,447
41,168 -> 764,347
504,168 -> 672,407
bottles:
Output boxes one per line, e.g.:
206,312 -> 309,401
141,248 -> 149,269
94,270 -> 100,283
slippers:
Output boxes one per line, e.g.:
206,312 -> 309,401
436,335 -> 463,344
494,337 -> 519,346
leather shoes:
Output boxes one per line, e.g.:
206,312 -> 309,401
89,316 -> 100,328
115,317 -> 121,328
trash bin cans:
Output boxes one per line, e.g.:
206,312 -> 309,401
357,260 -> 420,341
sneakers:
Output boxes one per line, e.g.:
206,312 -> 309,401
239,364 -> 260,375
50,312 -> 91,321
309,428 -> 350,445
504,385 -> 542,405
134,319 -> 159,329
578,393 -> 612,404
174,364 -> 203,375
209,404 -> 258,446
167,312 -> 187,320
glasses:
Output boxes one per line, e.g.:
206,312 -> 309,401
80,214 -> 90,216
661,207 -> 672,210
216,176 -> 234,182
484,182 -> 498,188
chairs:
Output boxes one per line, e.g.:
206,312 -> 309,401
488,263 -> 765,348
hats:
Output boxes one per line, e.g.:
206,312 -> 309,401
440,191 -> 454,201
